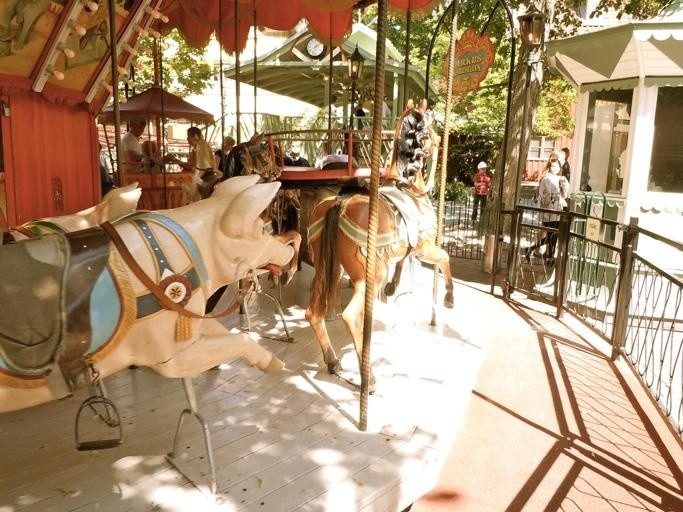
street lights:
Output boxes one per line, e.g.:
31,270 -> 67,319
500,0 -> 548,290
341,40 -> 366,132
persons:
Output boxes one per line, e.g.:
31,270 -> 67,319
470,161 -> 492,226
353,94 -> 440,194
524,159 -> 570,267
98,116 -> 332,201
537,148 -> 570,208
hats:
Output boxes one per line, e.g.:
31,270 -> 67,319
477,161 -> 488,169
